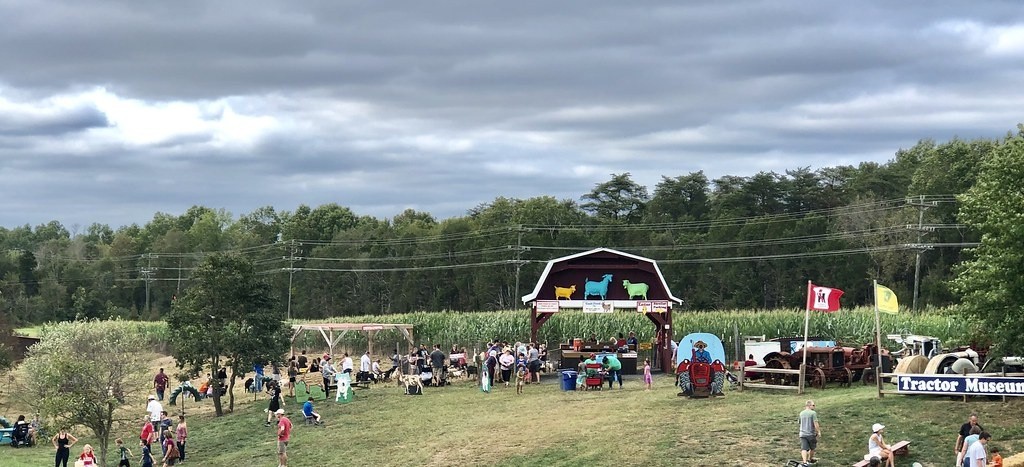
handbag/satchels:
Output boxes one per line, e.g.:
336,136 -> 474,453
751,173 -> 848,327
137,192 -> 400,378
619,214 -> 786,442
168,448 -> 180,459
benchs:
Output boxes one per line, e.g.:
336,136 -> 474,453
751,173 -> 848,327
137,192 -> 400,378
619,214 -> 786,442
328,381 -> 370,389
852,441 -> 910,467
302,411 -> 316,426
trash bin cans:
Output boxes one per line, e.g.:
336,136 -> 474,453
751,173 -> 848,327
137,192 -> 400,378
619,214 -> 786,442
562,371 -> 577,390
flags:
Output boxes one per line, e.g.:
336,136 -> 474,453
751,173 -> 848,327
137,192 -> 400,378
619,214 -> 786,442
809,283 -> 843,311
876,282 -> 899,316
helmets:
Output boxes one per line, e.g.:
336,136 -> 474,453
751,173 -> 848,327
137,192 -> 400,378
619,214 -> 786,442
590,353 -> 596,359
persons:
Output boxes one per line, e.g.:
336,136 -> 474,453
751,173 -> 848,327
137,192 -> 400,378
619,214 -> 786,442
274,409 -> 293,467
10,413 -> 41,448
745,354 -> 757,379
955,416 -> 1002,467
869,423 -> 895,467
139,368 -> 227,467
52,426 -> 78,467
80,444 -> 96,467
798,400 -> 821,467
114,439 -> 134,467
576,331 -> 652,391
252,337 -> 547,427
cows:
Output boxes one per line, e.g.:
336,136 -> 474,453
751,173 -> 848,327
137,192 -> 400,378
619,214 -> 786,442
393,371 -> 423,395
447,365 -> 465,382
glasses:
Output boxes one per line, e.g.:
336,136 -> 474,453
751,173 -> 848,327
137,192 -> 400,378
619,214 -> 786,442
811,405 -> 816,407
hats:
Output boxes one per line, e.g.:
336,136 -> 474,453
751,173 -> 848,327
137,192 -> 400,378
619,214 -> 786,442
619,332 -> 623,338
148,395 -> 157,399
694,341 -> 708,349
504,347 -> 512,353
274,409 -> 285,414
518,346 -> 526,353
610,337 -> 615,343
326,358 -> 331,361
873,423 -> 885,432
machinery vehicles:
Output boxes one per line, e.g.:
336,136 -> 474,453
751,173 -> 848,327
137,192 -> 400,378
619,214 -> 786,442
763,340 -> 882,390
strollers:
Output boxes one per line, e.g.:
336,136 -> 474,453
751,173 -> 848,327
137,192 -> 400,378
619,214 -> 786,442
428,359 -> 451,386
10,423 -> 33,448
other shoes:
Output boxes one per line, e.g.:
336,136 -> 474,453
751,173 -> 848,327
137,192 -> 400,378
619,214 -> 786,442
264,422 -> 270,426
807,459 -> 817,463
